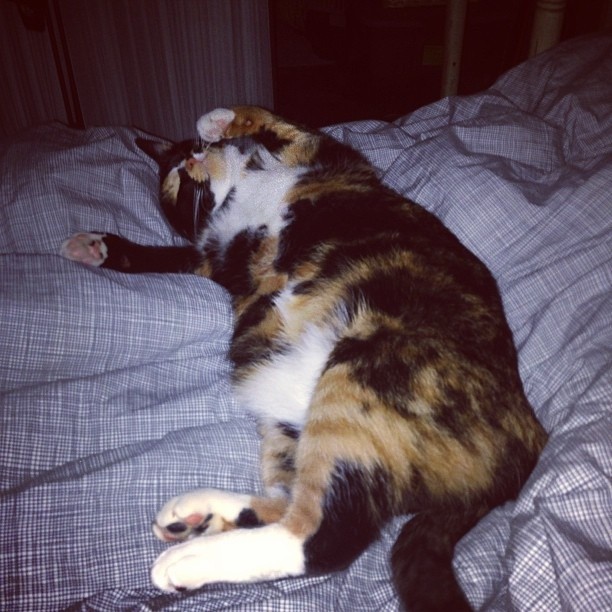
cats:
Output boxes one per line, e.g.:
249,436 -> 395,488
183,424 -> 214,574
57,106 -> 549,612
